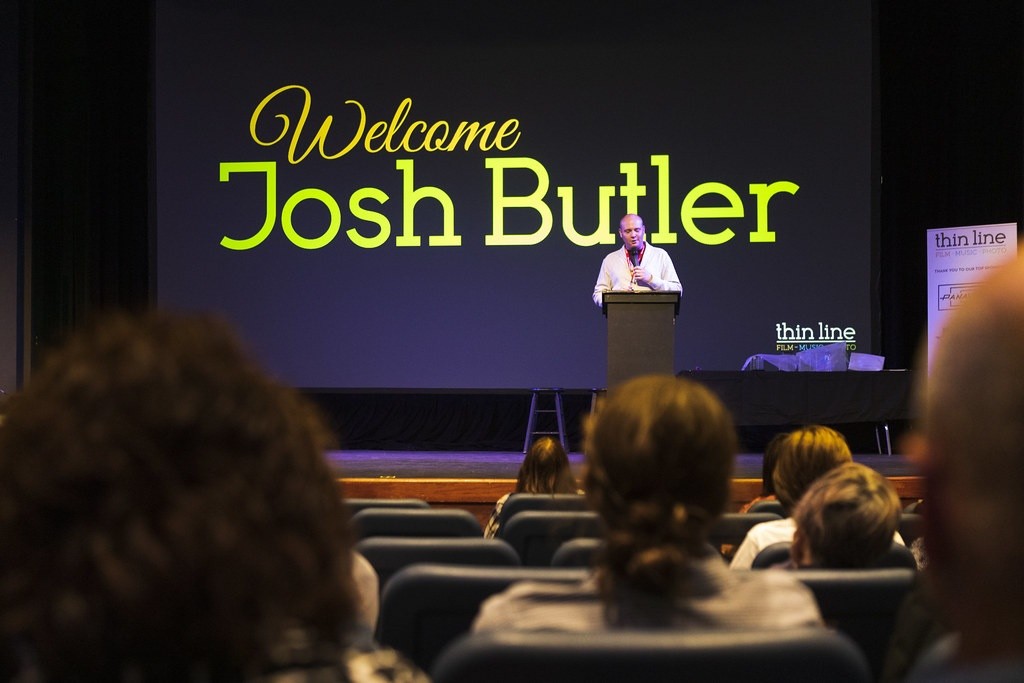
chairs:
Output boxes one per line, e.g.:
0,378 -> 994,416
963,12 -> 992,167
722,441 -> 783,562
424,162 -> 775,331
305,477 -> 938,683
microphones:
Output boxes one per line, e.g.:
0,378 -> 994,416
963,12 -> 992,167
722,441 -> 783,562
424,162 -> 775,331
630,246 -> 640,268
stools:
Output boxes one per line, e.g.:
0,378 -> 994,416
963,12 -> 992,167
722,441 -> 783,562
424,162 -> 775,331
586,387 -> 609,456
524,385 -> 570,456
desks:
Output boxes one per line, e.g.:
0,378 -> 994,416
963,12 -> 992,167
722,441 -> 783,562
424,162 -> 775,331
674,366 -> 911,455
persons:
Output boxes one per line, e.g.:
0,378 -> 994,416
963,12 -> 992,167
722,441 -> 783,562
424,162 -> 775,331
0,289 -> 434,683
888,238 -> 1024,683
726,422 -> 855,569
719,432 -> 793,560
908,536 -> 930,570
763,459 -> 905,570
482,437 -> 588,539
469,372 -> 823,642
346,545 -> 384,645
591,214 -> 683,311
902,498 -> 925,514
